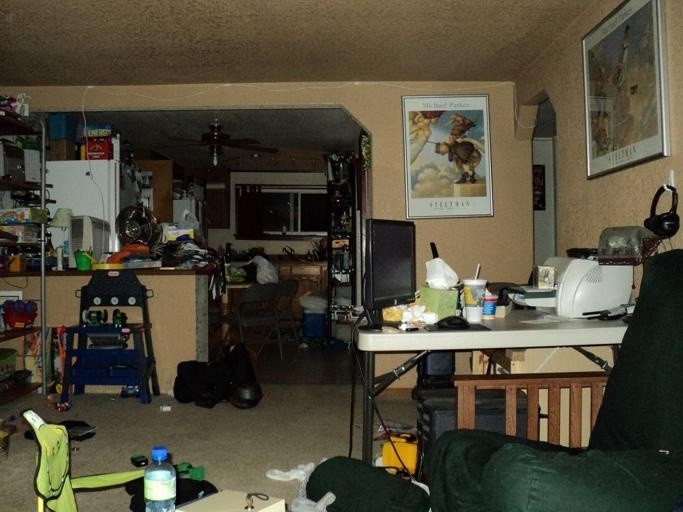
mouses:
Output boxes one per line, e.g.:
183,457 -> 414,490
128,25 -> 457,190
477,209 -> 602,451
436,315 -> 469,328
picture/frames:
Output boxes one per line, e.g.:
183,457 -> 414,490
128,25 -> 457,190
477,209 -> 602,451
399,93 -> 495,221
578,0 -> 670,182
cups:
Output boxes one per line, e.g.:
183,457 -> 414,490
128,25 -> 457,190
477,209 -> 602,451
462,279 -> 485,322
478,295 -> 496,319
75,250 -> 95,271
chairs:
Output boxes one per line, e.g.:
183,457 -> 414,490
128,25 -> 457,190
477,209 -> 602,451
416,248 -> 680,512
221,276 -> 304,361
22,409 -> 146,511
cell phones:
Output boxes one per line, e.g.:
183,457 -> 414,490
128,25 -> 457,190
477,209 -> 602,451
131,454 -> 147,467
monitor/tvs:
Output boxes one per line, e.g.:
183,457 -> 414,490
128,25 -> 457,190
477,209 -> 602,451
356,219 -> 415,329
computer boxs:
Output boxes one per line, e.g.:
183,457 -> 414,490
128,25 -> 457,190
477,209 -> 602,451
410,388 -> 540,476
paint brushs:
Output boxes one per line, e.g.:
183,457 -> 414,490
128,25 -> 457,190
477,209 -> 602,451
405,328 -> 419,331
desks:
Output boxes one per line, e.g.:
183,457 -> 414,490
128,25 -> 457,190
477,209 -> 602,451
345,310 -> 627,459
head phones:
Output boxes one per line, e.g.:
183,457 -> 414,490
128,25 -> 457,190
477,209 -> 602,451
645,184 -> 680,238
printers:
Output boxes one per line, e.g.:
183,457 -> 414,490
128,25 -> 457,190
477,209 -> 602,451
507,255 -> 635,317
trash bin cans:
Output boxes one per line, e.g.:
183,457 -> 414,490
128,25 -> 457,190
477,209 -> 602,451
303,310 -> 325,337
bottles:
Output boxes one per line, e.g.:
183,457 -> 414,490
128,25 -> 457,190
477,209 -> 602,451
143,446 -> 176,511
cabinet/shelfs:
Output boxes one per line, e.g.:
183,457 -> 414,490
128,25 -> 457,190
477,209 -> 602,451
0,114 -> 49,406
59,321 -> 151,406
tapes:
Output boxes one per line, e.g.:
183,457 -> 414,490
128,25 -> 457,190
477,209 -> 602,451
90,264 -> 125,270
